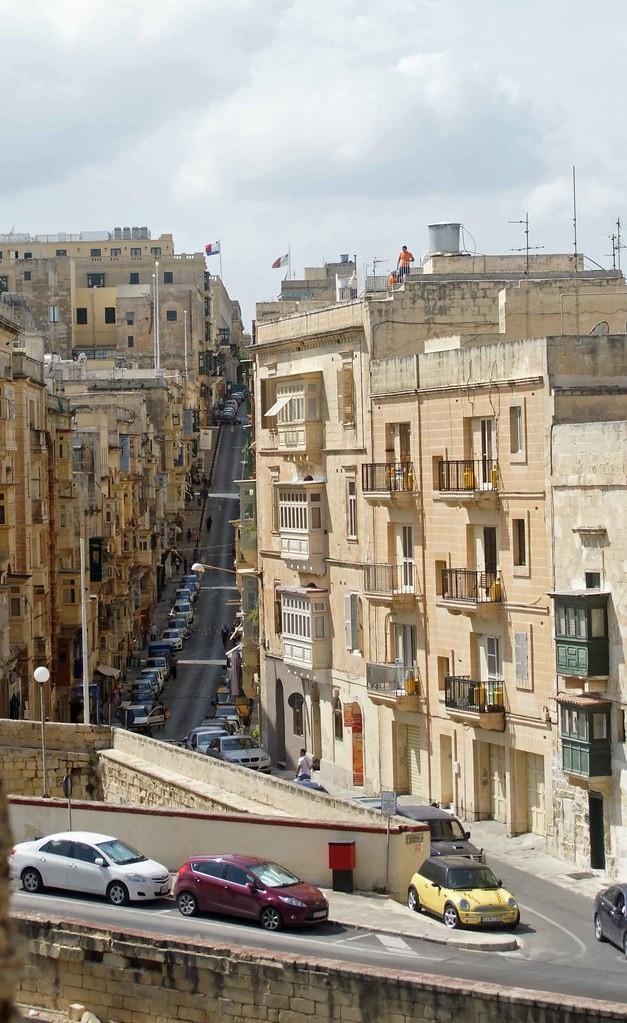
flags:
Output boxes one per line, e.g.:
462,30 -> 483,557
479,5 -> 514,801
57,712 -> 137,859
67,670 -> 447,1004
271,254 -> 289,269
204,240 -> 220,256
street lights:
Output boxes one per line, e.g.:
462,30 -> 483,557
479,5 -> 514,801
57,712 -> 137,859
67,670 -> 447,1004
192,562 -> 265,645
34,667 -> 51,799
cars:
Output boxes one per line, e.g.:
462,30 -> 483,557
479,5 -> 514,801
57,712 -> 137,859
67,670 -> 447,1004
407,855 -> 520,931
112,657 -> 244,756
174,853 -> 329,931
594,882 -> 627,958
205,735 -> 271,774
157,574 -> 201,650
9,830 -> 173,906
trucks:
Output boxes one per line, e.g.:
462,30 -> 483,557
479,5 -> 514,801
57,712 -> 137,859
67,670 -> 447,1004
148,641 -> 176,674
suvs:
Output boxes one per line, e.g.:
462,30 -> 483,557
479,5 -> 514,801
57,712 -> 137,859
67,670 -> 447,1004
393,805 -> 486,865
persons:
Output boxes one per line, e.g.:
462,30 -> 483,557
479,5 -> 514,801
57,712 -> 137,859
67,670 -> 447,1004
187,471 -> 210,514
175,556 -> 181,574
220,624 -> 229,648
387,270 -> 400,287
205,516 -> 213,531
396,245 -> 415,278
183,556 -> 188,575
295,749 -> 314,781
157,702 -> 167,728
187,527 -> 193,545
171,654 -> 178,680
149,623 -> 158,642
